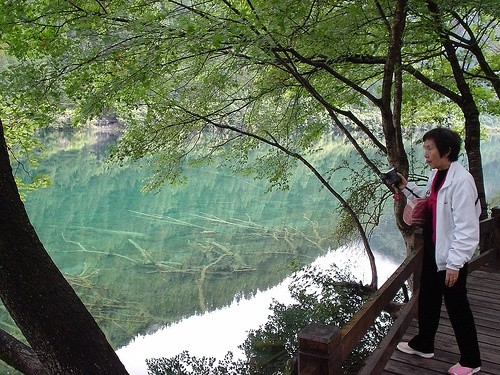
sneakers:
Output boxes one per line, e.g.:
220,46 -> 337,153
396,341 -> 435,358
447,362 -> 481,375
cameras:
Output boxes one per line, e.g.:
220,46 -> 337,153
379,167 -> 400,186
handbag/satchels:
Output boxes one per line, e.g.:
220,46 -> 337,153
402,199 -> 429,226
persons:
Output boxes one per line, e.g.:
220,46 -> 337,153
391,126 -> 482,375
